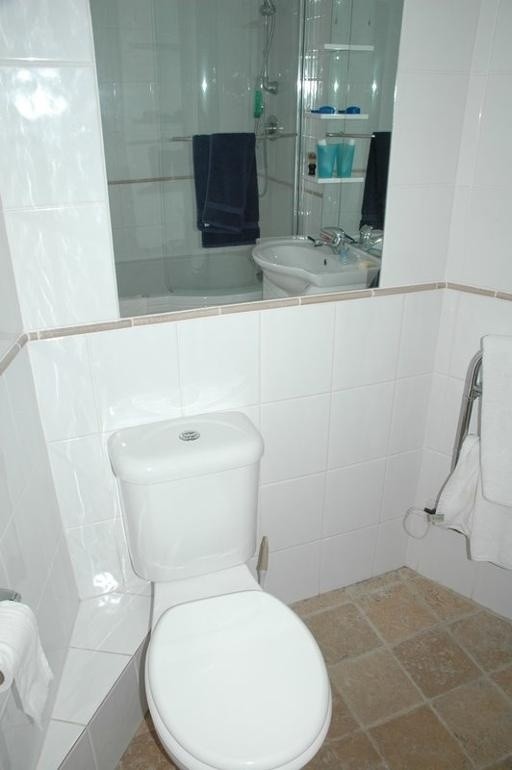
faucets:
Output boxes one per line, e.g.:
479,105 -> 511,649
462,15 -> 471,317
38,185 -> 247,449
359,224 -> 383,248
309,229 -> 350,256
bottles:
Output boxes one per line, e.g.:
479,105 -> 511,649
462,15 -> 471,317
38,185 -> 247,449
315,141 -> 355,178
307,151 -> 317,176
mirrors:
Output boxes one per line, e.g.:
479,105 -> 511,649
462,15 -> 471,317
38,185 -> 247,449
293,0 -> 402,263
89,0 -> 402,320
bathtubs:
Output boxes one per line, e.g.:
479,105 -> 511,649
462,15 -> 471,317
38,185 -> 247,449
117,240 -> 284,315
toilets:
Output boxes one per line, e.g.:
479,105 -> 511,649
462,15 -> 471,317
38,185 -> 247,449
107,410 -> 333,767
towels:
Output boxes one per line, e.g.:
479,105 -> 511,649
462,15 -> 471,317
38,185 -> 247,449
429,330 -> 512,573
190,133 -> 262,249
359,132 -> 392,231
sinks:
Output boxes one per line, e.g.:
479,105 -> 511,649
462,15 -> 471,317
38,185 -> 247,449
251,234 -> 379,297
